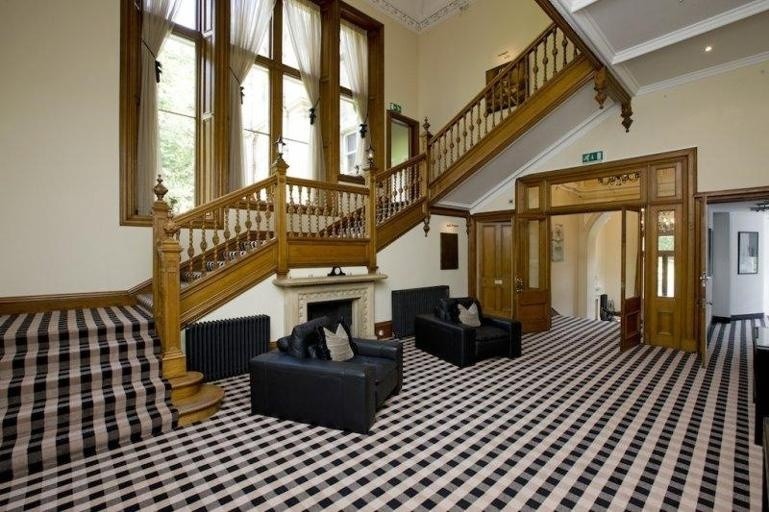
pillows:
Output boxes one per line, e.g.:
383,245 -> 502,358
277,314 -> 359,364
435,296 -> 485,328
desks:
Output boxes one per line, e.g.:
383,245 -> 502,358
750,325 -> 769,445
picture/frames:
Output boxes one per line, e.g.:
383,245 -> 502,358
738,231 -> 760,275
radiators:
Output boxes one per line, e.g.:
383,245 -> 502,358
186,314 -> 272,383
391,284 -> 450,338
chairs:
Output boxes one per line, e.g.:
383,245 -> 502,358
248,337 -> 404,434
413,313 -> 523,368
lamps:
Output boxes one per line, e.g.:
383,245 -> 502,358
365,144 -> 376,168
273,134 -> 288,161
